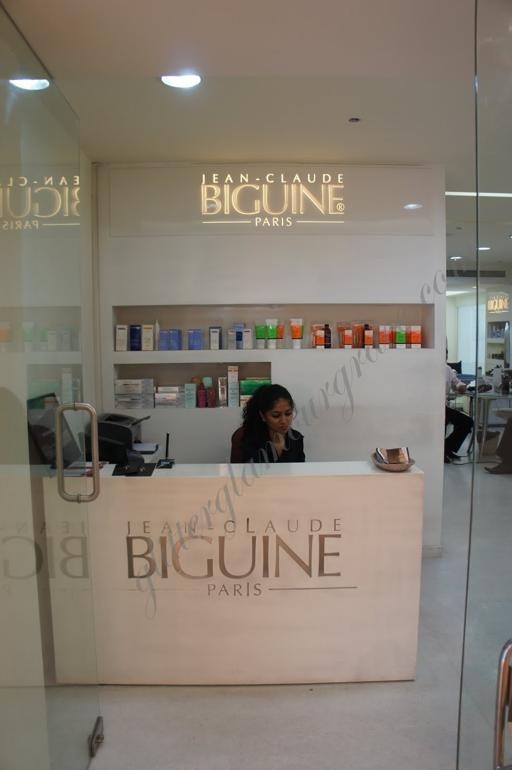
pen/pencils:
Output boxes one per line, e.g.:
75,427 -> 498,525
166,432 -> 169,459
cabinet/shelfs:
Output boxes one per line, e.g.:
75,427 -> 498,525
484,319 -> 509,376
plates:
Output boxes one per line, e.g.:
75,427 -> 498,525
370,453 -> 415,472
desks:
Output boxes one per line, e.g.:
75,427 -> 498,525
454,390 -> 512,463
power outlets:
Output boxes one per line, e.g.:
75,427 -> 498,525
89,716 -> 106,758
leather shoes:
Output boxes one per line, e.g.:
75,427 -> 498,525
484,464 -> 512,473
445,451 -> 461,463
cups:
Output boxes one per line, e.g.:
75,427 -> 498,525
386,447 -> 411,464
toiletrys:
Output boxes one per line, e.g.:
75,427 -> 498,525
198,383 -> 206,407
277,323 -> 284,349
325,324 -> 331,348
363,324 -> 369,348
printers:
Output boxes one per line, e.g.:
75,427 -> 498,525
85,413 -> 159,463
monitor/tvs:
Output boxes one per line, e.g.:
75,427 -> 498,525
26,392 -> 82,478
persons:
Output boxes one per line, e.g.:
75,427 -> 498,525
446,348 -> 474,463
230,384 -> 305,463
485,417 -> 511,474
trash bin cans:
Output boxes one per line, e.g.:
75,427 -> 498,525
477,430 -> 501,457
